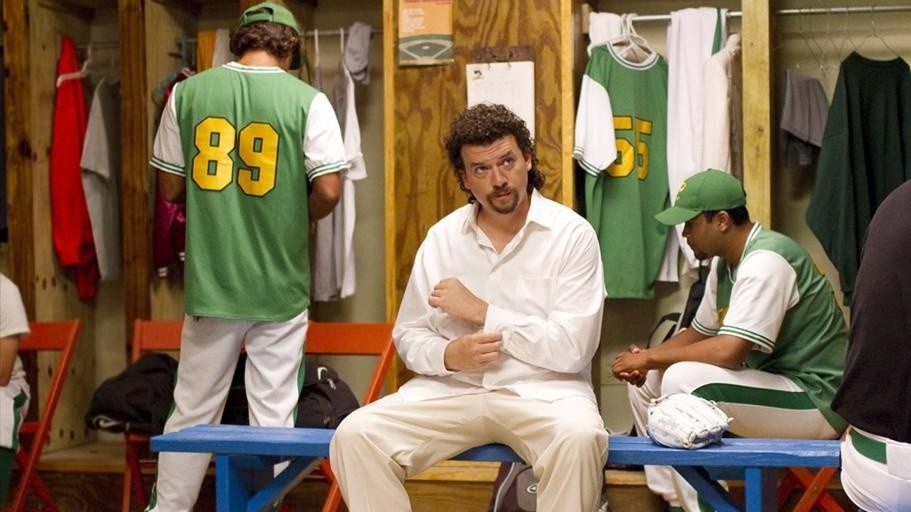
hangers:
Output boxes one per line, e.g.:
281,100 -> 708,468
769,4 -> 901,77
53,39 -> 108,89
601,6 -> 738,62
174,22 -> 350,76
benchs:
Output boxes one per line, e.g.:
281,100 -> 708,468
140,419 -> 847,511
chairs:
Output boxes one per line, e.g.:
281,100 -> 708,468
287,318 -> 397,512
6,313 -> 84,512
113,308 -> 217,510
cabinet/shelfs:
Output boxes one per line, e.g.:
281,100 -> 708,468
0,1 -> 395,482
563,2 -> 910,487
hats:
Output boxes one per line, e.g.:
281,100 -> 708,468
654,168 -> 746,226
235,2 -> 300,70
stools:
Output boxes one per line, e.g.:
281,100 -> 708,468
775,431 -> 847,512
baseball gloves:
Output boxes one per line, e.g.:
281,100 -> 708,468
646,389 -> 735,448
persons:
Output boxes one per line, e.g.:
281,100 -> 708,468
332,101 -> 611,512
0,264 -> 34,512
144,2 -> 348,512
611,168 -> 851,512
830,178 -> 910,512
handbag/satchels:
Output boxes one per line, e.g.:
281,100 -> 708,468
293,361 -> 359,428
